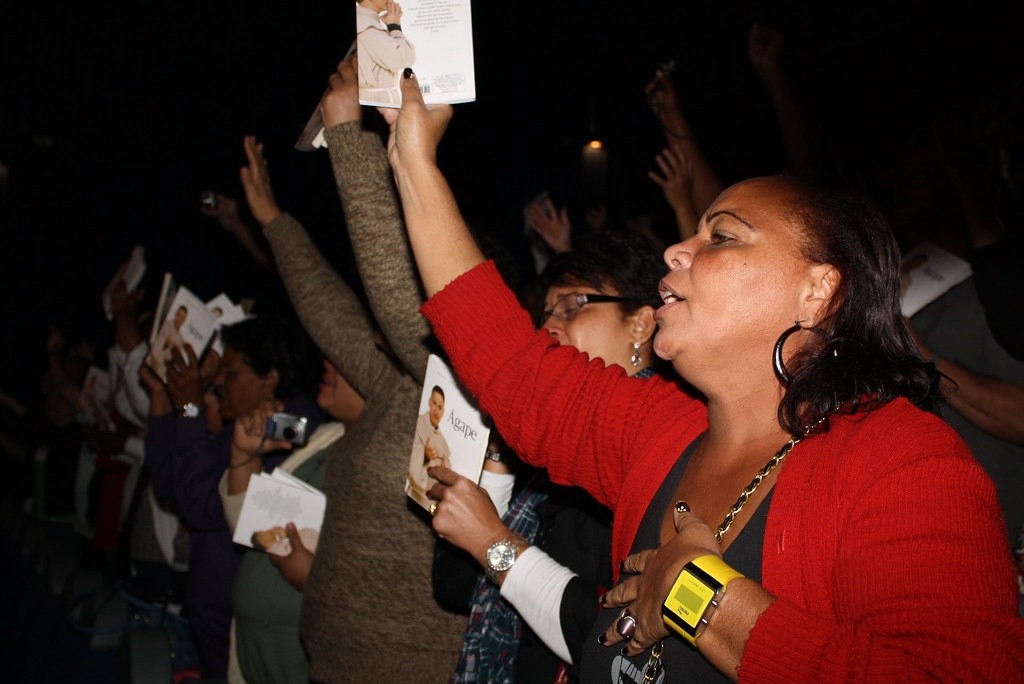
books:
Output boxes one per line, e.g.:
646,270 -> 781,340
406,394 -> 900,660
206,293 -> 243,356
145,287 -> 217,384
230,473 -> 329,556
294,41 -> 356,152
405,353 -> 491,519
355,0 -> 476,107
896,240 -> 971,320
79,366 -> 109,416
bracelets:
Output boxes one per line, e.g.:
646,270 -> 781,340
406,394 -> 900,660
485,449 -> 500,462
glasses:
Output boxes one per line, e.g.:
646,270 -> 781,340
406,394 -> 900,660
537,291 -> 635,324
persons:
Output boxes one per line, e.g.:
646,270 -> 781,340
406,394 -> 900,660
34,21 -> 1024,684
211,308 -> 222,318
356,0 -> 418,105
149,305 -> 188,373
408,385 -> 450,515
250,527 -> 320,557
899,253 -> 926,295
82,374 -> 97,406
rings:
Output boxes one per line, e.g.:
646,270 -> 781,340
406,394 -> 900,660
617,611 -> 638,641
428,503 -> 437,513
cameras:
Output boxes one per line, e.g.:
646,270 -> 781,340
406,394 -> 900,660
265,412 -> 310,445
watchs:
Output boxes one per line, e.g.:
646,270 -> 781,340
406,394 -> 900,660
485,536 -> 524,583
660,555 -> 750,646
179,402 -> 199,419
